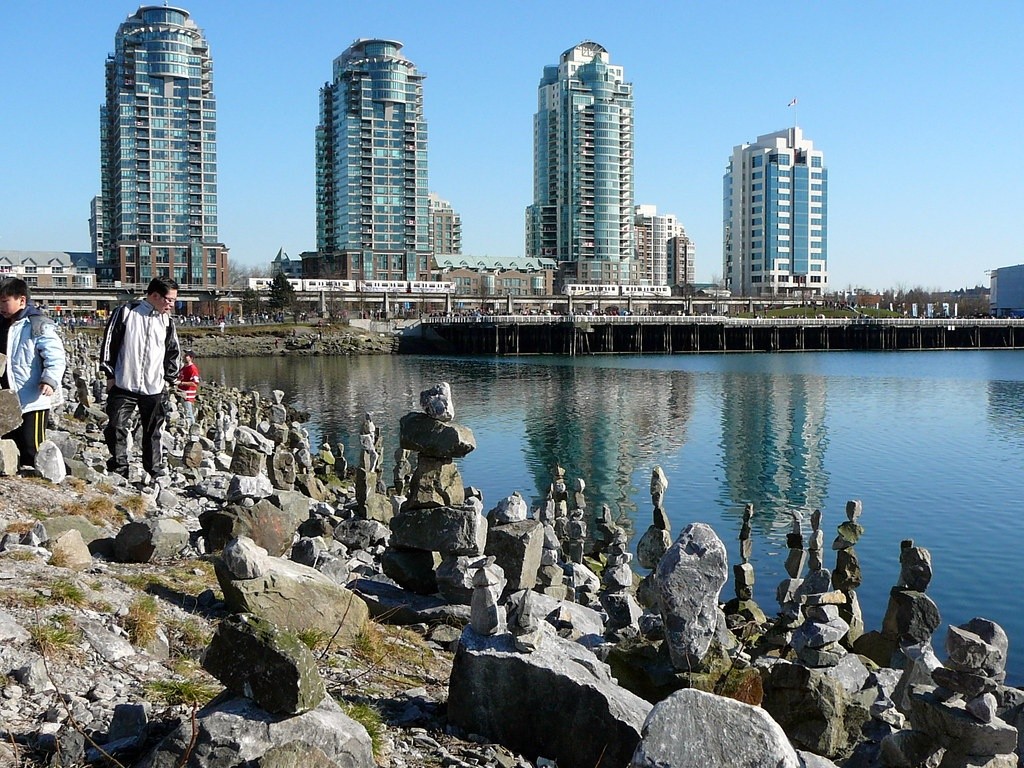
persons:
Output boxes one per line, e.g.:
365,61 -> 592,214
174,305 -> 423,326
429,305 -> 747,319
50,315 -> 110,333
0,277 -> 66,466
174,350 -> 199,425
755,300 -> 1024,324
100,276 -> 181,479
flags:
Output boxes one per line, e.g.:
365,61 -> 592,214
788,98 -> 797,107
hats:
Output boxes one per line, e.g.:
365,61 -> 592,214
183,349 -> 196,359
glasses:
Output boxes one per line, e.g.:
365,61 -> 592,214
156,290 -> 176,304
